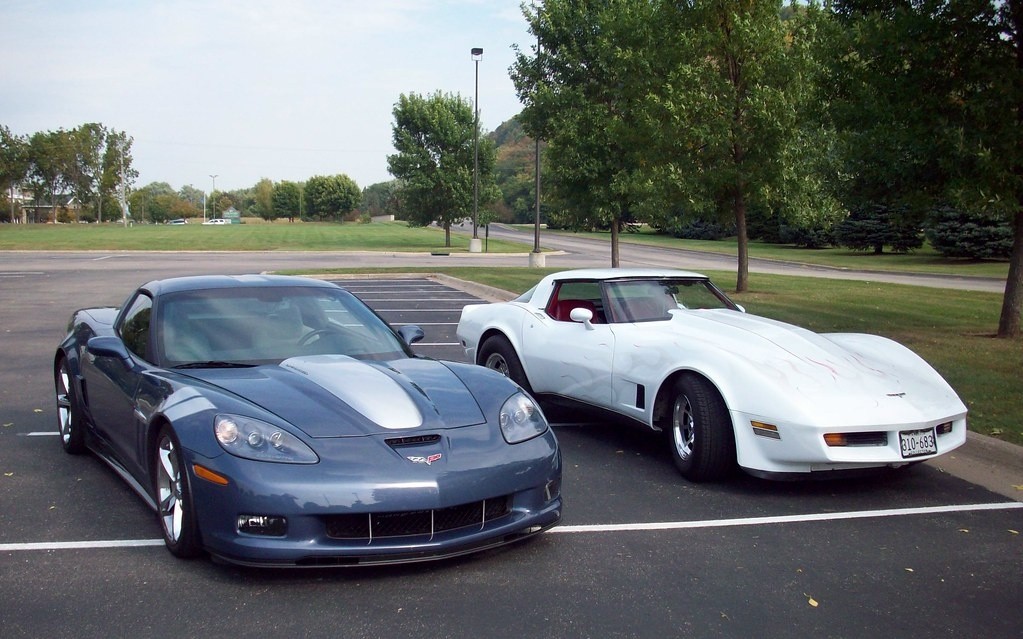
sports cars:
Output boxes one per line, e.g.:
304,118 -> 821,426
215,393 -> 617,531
53,271 -> 568,573
454,266 -> 971,489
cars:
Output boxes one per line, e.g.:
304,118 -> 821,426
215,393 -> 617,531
169,219 -> 184,227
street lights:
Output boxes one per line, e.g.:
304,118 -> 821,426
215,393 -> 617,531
209,174 -> 219,219
471,47 -> 485,253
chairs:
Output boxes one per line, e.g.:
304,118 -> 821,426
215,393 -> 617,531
556,299 -> 598,324
252,302 -> 320,349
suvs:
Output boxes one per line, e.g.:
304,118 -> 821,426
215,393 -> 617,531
201,219 -> 226,225
